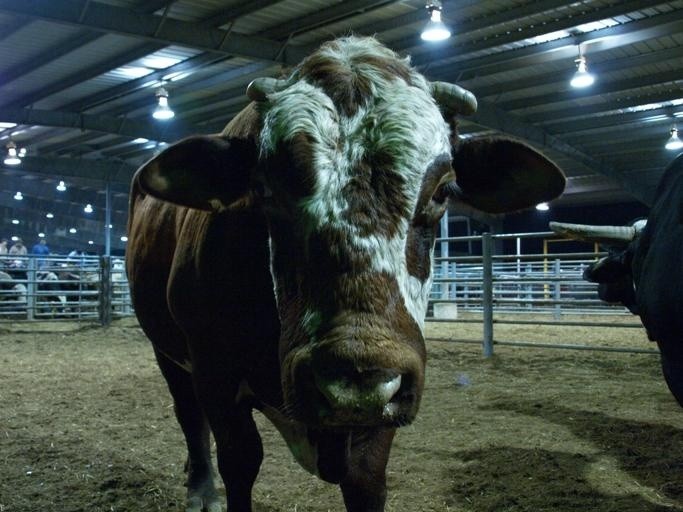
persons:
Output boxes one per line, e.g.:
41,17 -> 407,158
9,238 -> 28,255
67,248 -> 89,265
0,239 -> 7,255
32,235 -> 51,266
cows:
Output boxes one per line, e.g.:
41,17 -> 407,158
549,154 -> 683,410
0,270 -> 115,320
125,30 -> 567,512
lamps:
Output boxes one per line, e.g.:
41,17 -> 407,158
571,44 -> 594,86
419,0 -> 451,40
151,87 -> 175,120
665,123 -> 683,149
